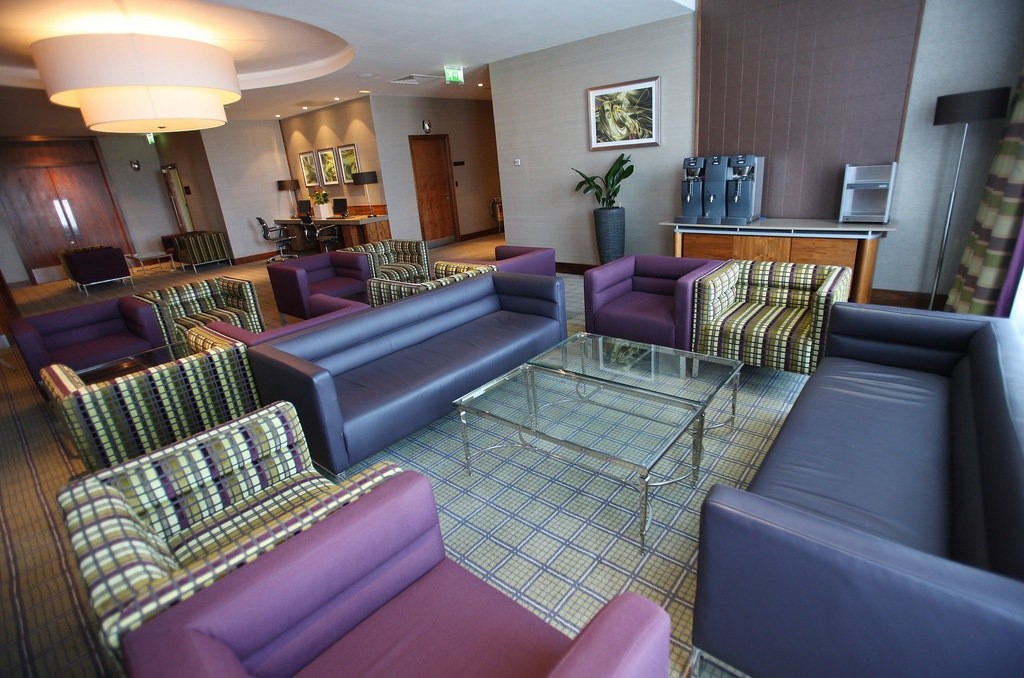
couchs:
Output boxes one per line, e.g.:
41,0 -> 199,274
8,240 -> 1024,678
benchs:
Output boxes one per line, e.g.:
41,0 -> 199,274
161,231 -> 232,273
58,245 -> 136,297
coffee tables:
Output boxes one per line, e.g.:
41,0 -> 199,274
452,362 -> 706,556
526,332 -> 744,481
126,251 -> 176,276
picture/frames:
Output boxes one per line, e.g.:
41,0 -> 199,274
317,147 -> 341,186
337,143 -> 361,184
587,76 -> 662,152
298,150 -> 321,188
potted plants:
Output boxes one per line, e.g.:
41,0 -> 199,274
310,190 -> 333,219
572,154 -> 634,265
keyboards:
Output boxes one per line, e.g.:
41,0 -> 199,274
326,217 -> 343,219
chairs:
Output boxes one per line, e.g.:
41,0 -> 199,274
299,215 -> 338,252
256,217 -> 299,263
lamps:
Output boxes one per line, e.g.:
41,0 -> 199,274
29,33 -> 242,133
278,179 -> 300,219
352,171 -> 378,218
928,86 -> 1012,310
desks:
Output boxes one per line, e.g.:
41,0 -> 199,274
657,218 -> 899,304
274,215 -> 392,255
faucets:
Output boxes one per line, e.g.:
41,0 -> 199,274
733,192 -> 740,203
707,190 -> 715,203
684,190 -> 692,204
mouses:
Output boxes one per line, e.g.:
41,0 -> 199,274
340,216 -> 345,219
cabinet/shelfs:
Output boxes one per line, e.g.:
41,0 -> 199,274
839,162 -> 897,224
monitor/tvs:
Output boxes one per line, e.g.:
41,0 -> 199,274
299,199 -> 311,212
333,199 -> 347,213
